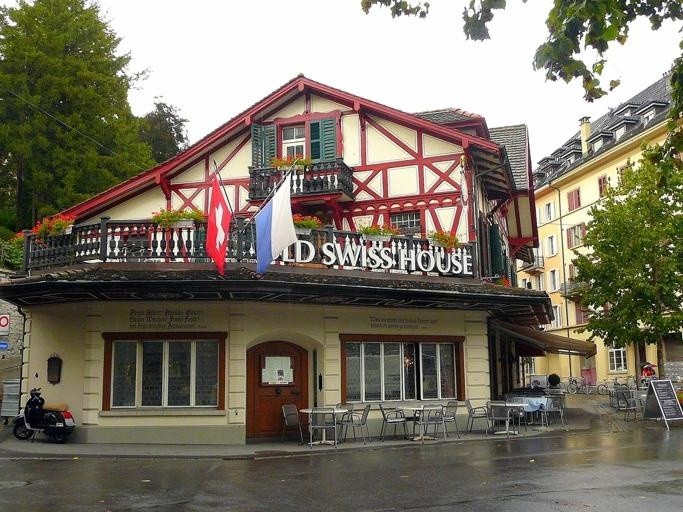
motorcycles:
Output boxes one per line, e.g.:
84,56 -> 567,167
12,387 -> 76,444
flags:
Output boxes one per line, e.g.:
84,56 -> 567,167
252,171 -> 297,274
204,171 -> 231,276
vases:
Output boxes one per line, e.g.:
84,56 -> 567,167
161,218 -> 195,228
295,227 -> 312,236
362,233 -> 393,242
434,239 -> 455,248
49,224 -> 74,236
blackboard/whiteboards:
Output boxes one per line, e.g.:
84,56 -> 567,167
650,380 -> 683,421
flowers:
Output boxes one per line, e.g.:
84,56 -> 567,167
433,230 -> 458,248
271,152 -> 310,165
362,223 -> 397,236
151,207 -> 204,228
292,213 -> 323,230
31,213 -> 77,234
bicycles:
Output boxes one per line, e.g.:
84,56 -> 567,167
597,380 -> 618,395
567,377 -> 592,394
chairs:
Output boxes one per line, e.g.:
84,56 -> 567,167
281,387 -> 568,449
612,378 -> 645,422
